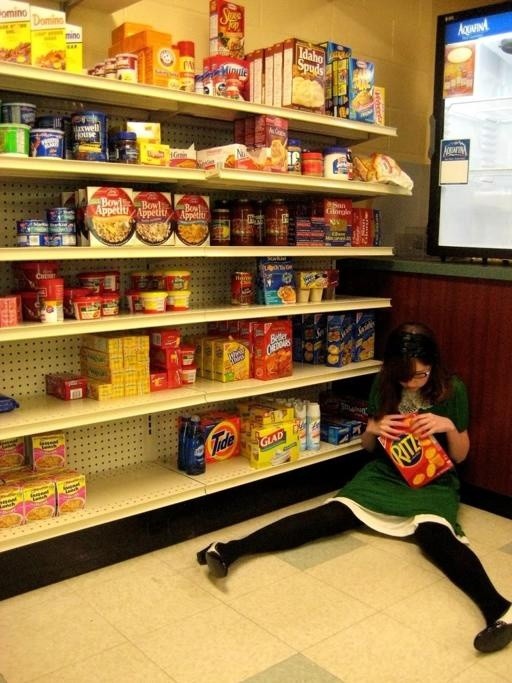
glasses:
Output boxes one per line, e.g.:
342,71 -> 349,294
412,364 -> 431,378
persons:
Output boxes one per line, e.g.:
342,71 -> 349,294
194,321 -> 512,659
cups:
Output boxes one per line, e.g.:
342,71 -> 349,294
296,288 -> 324,302
64,266 -> 191,319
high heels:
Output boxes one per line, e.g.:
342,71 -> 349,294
474,602 -> 512,652
197,541 -> 228,577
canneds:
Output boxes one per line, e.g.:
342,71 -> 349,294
88,53 -> 138,85
287,138 -> 324,177
230,271 -> 257,306
15,207 -> 77,247
71,110 -> 141,165
210,197 -> 290,246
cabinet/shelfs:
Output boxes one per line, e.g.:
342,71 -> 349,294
1,58 -> 414,554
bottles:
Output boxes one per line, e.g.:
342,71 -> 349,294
177,411 -> 205,476
178,40 -> 196,91
264,395 -> 320,452
346,149 -> 353,179
194,69 -> 239,98
